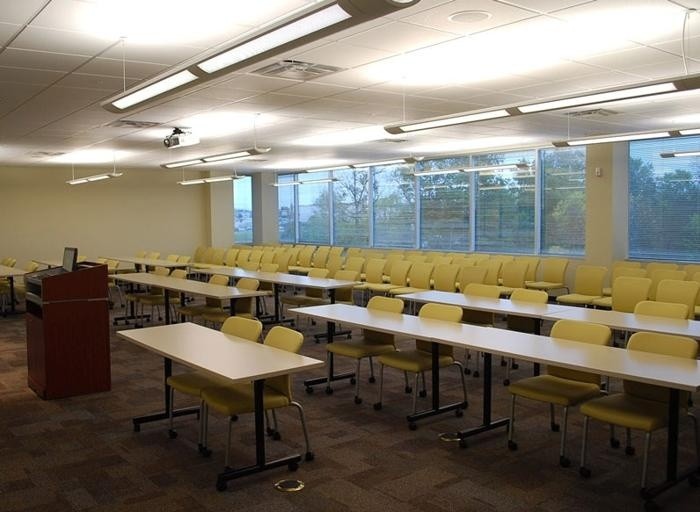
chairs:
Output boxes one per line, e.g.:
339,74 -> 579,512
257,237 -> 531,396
325,296 -> 412,405
508,319 -> 620,467
373,302 -> 469,422
579,332 -> 700,501
202,326 -> 314,473
166,316 -> 273,453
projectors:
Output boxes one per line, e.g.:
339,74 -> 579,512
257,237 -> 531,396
163,126 -> 200,149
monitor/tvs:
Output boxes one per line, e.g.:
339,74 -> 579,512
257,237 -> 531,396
61,247 -> 77,273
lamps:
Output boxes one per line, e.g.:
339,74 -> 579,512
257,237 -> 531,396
383,73 -> 700,135
423,152 -> 699,190
100,1 -> 420,113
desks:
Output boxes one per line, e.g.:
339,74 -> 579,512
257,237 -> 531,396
288,304 -> 700,511
115,322 -> 325,492
